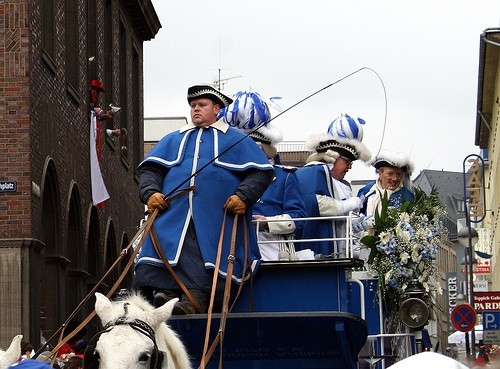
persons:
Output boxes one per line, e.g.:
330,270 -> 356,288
245,125 -> 309,261
357,151 -> 415,270
473,343 -> 500,369
136,85 -> 277,312
0,322 -> 99,369
295,136 -> 363,260
88,79 -> 127,152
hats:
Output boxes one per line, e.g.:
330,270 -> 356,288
305,113 -> 372,164
187,84 -> 233,110
365,148 -> 416,191
217,88 -> 283,145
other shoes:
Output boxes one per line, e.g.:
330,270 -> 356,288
172,302 -> 196,314
154,293 -> 170,308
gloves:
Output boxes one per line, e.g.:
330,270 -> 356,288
224,195 -> 246,215
147,193 -> 169,216
341,196 -> 363,215
349,211 -> 370,233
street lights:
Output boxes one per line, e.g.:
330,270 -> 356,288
456,225 -> 480,358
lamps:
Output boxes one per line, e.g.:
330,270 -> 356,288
94,103 -> 122,121
106,127 -> 128,152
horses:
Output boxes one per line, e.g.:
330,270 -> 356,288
81,290 -> 194,369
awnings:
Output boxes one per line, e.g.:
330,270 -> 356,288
447,324 -> 483,345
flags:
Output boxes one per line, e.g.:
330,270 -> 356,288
91,110 -> 112,209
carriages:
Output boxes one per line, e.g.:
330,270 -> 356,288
0,209 -> 435,369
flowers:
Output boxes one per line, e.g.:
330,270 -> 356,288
360,182 -> 450,308
